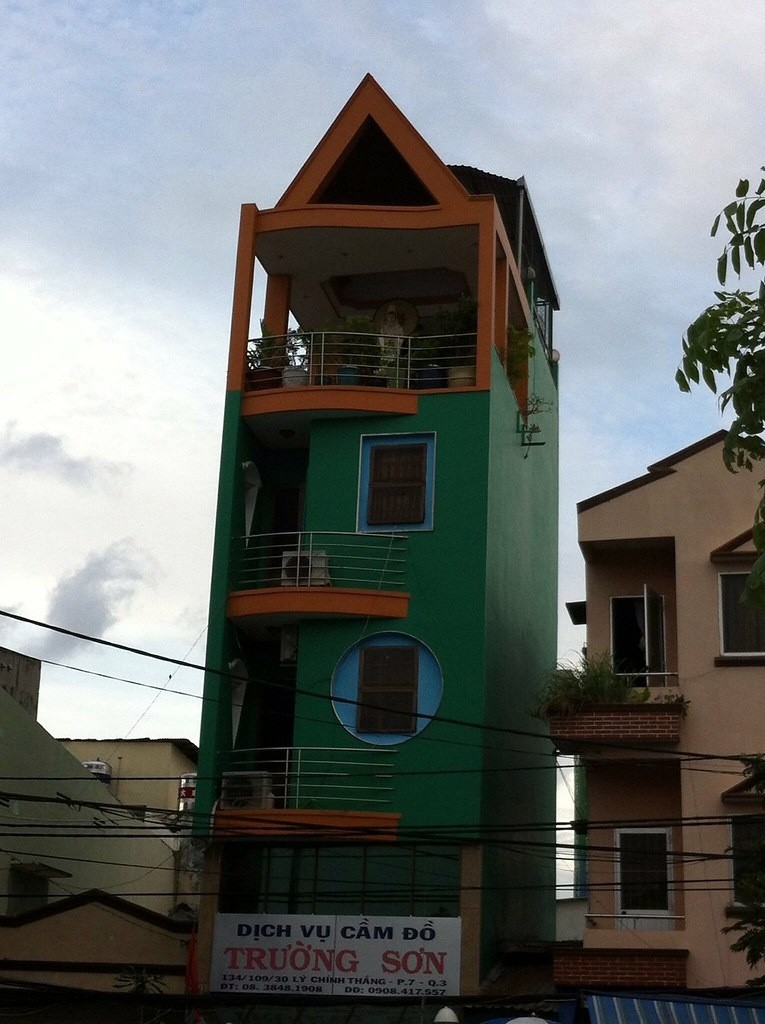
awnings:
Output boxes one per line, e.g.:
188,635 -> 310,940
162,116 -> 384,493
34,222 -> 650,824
585,991 -> 764,1024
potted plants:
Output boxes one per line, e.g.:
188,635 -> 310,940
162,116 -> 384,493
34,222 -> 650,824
244,293 -> 477,390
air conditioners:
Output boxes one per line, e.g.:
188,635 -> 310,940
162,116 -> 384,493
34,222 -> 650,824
281,550 -> 327,585
221,772 -> 273,810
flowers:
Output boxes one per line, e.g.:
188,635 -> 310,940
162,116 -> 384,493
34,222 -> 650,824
516,393 -> 552,415
522,424 -> 541,459
